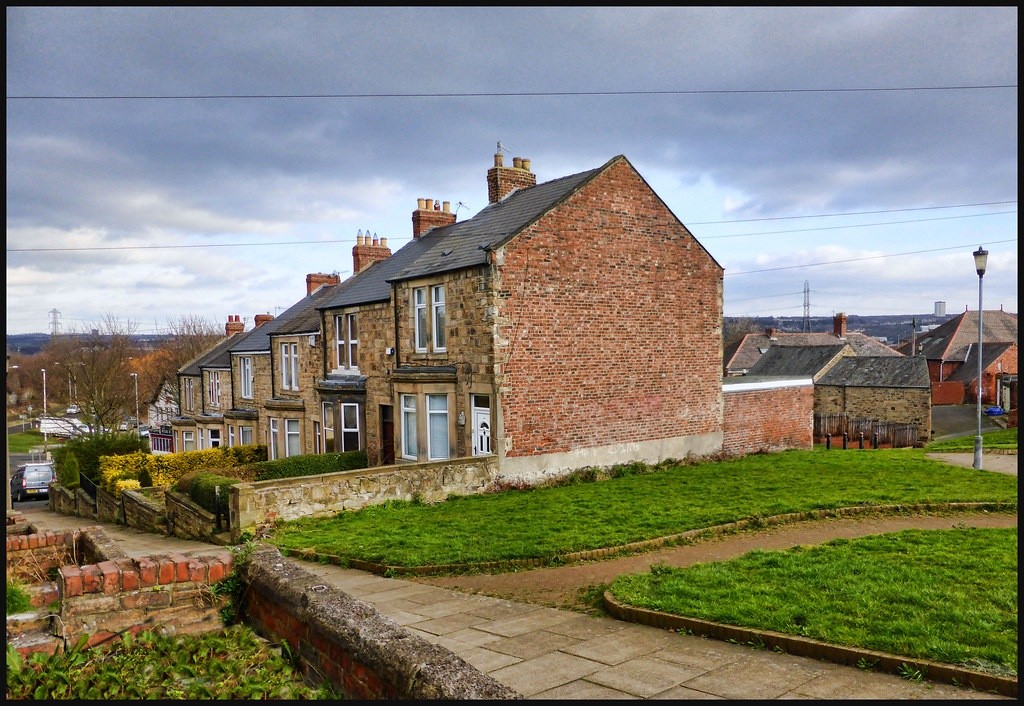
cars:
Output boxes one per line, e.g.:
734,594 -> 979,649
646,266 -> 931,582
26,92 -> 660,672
67,405 -> 81,414
93,417 -> 149,438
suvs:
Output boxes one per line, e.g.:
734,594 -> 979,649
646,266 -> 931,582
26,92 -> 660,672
10,463 -> 58,502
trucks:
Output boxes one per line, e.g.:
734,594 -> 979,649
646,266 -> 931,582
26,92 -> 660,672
40,417 -> 90,439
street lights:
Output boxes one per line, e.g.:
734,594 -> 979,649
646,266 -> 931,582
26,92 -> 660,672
41,369 -> 47,444
973,246 -> 989,470
130,372 -> 139,440
